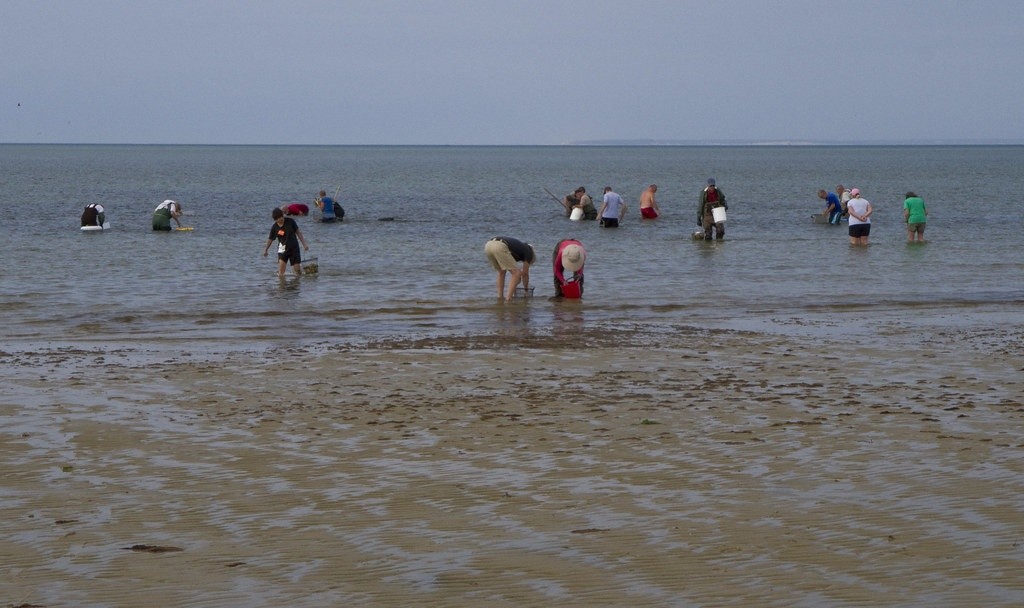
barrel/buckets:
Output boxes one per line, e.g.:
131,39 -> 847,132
560,277 -> 581,298
711,206 -> 727,224
300,250 -> 320,279
569,207 -> 584,221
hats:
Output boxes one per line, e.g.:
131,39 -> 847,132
522,244 -> 536,264
707,178 -> 716,187
849,188 -> 860,196
561,244 -> 585,271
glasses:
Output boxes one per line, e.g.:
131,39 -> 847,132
711,185 -> 714,187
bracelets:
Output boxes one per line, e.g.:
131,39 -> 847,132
305,247 -> 308,250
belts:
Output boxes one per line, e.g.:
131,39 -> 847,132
490,238 -> 510,247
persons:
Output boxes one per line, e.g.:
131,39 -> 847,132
280,203 -> 309,216
903,191 -> 928,241
81,203 -> 105,227
484,236 -> 536,301
640,184 -> 660,220
552,239 -> 586,298
597,186 -> 628,228
315,191 -> 346,224
565,186 -> 598,221
264,207 -> 309,275
152,200 -> 183,231
697,177 -> 728,240
818,185 -> 872,245
